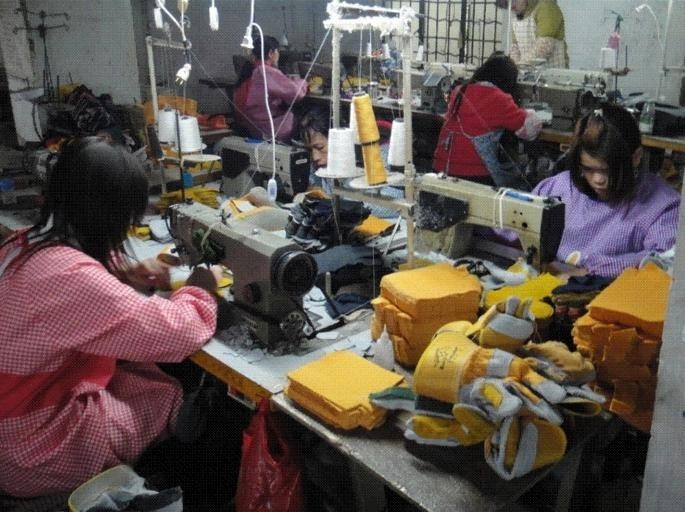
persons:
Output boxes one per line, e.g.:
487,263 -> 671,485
429,50 -> 547,194
494,0 -> 570,70
232,34 -> 308,143
287,103 -> 431,219
1,134 -> 239,511
484,102 -> 682,281
